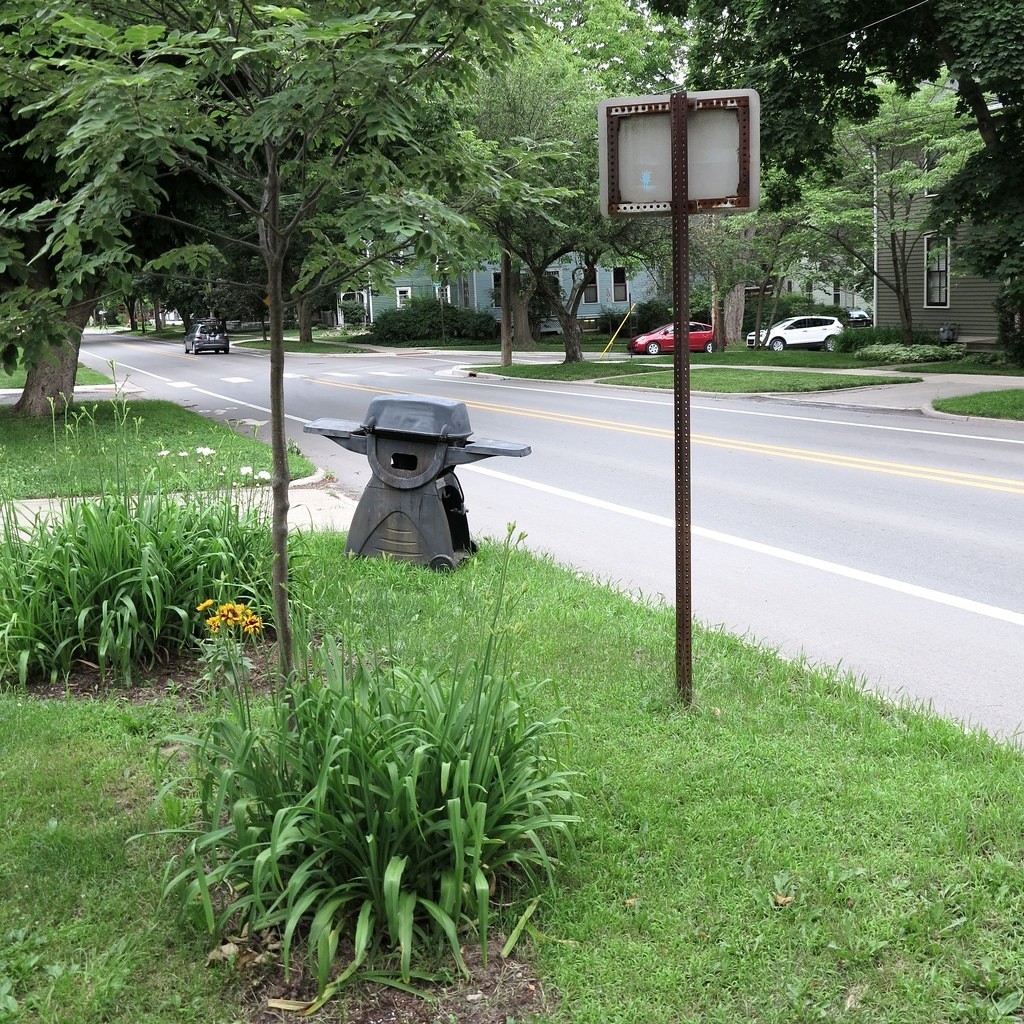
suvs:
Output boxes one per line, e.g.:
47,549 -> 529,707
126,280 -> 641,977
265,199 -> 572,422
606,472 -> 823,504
183,318 -> 230,355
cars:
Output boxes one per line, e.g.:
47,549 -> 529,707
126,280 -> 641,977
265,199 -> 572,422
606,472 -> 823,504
746,315 -> 845,351
628,321 -> 728,355
821,306 -> 873,331
